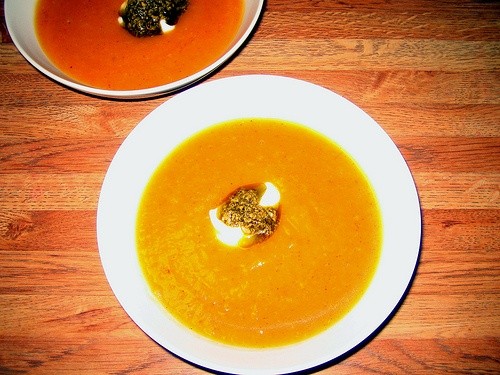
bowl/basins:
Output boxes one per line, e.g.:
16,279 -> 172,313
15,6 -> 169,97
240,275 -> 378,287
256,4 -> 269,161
2,0 -> 265,99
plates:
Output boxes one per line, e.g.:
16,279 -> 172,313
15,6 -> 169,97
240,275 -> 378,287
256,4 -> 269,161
95,74 -> 422,375
